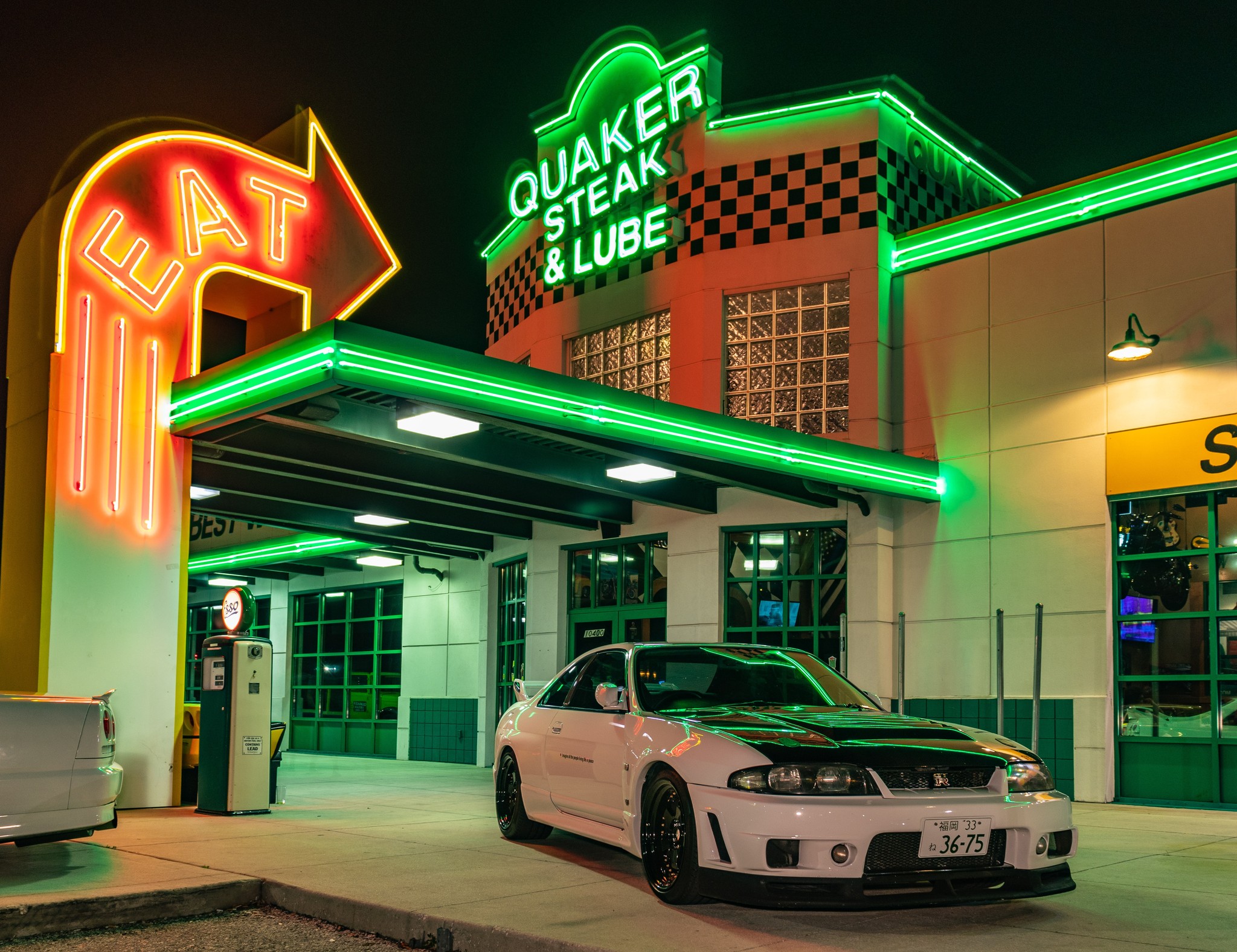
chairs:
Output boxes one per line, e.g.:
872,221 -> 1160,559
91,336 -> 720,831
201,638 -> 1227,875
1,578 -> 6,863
727,660 -> 777,703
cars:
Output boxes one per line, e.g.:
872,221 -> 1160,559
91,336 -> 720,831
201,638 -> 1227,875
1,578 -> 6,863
492,641 -> 1077,907
1,689 -> 127,845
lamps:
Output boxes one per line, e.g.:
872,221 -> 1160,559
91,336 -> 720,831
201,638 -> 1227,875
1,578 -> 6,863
352,511 -> 410,528
393,401 -> 482,442
190,485 -> 221,501
602,456 -> 677,484
355,550 -> 404,568
587,550 -> 636,564
208,573 -> 249,587
1107,312 -> 1159,362
